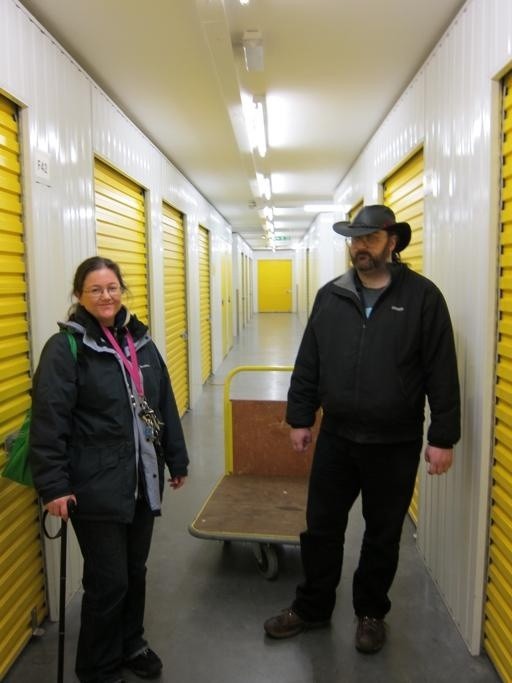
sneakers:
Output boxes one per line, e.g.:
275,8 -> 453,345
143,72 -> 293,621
351,613 -> 390,656
261,602 -> 334,641
126,645 -> 164,679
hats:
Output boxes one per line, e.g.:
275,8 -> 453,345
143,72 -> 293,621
330,204 -> 413,256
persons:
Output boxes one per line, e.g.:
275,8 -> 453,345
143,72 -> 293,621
22,254 -> 192,681
258,203 -> 463,655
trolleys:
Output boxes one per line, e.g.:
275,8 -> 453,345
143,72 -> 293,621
188,364 -> 325,581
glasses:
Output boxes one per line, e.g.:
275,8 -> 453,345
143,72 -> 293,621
78,285 -> 123,297
345,234 -> 390,246
